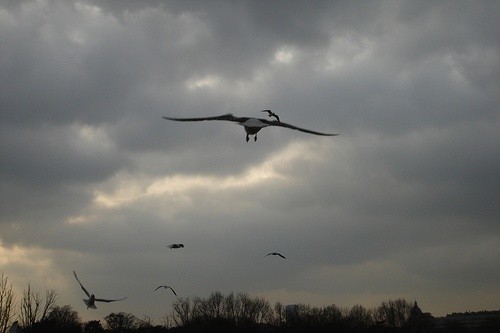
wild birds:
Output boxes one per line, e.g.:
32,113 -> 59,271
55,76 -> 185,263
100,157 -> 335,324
261,109 -> 280,122
167,243 -> 184,249
162,114 -> 341,143
154,286 -> 177,296
265,253 -> 286,258
73,270 -> 128,310
